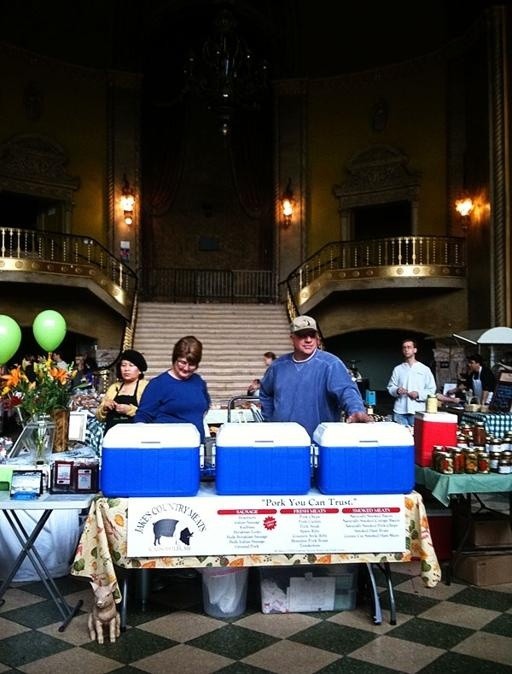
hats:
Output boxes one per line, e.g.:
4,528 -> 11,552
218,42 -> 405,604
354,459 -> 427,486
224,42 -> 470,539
122,349 -> 147,372
289,316 -> 318,334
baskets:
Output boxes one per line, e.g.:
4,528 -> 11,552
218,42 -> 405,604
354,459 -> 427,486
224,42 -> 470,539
465,397 -> 481,412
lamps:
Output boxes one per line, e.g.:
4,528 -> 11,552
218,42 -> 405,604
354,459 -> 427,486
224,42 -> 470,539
117,175 -> 138,225
280,179 -> 295,228
182,1 -> 272,139
454,188 -> 476,231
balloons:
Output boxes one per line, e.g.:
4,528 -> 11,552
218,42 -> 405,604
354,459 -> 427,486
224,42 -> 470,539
0,313 -> 22,365
32,308 -> 67,353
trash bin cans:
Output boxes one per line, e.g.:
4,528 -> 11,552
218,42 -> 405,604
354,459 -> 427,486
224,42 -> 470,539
196,567 -> 249,618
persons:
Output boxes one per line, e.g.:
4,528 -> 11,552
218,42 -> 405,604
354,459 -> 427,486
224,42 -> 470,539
132,334 -> 212,445
252,378 -> 263,397
258,315 -> 377,444
435,392 -> 464,403
386,337 -> 437,426
95,349 -> 150,440
445,354 -> 498,406
263,351 -> 275,366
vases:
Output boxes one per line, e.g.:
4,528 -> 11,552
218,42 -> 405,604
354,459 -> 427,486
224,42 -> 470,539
26,414 -> 57,463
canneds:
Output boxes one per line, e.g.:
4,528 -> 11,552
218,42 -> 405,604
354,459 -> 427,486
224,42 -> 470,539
432,421 -> 512,474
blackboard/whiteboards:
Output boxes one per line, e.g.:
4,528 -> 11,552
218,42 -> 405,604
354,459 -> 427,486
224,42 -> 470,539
488,380 -> 512,414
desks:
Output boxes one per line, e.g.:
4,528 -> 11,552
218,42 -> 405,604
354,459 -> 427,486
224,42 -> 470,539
417,399 -> 512,587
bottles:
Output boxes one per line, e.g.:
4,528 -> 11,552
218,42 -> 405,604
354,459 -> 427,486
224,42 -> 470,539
432,421 -> 512,474
425,395 -> 438,413
35,461 -> 48,492
340,410 -> 346,422
367,406 -> 392,423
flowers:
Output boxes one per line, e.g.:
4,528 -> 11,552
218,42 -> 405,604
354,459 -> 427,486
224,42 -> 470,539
1,355 -> 82,457
